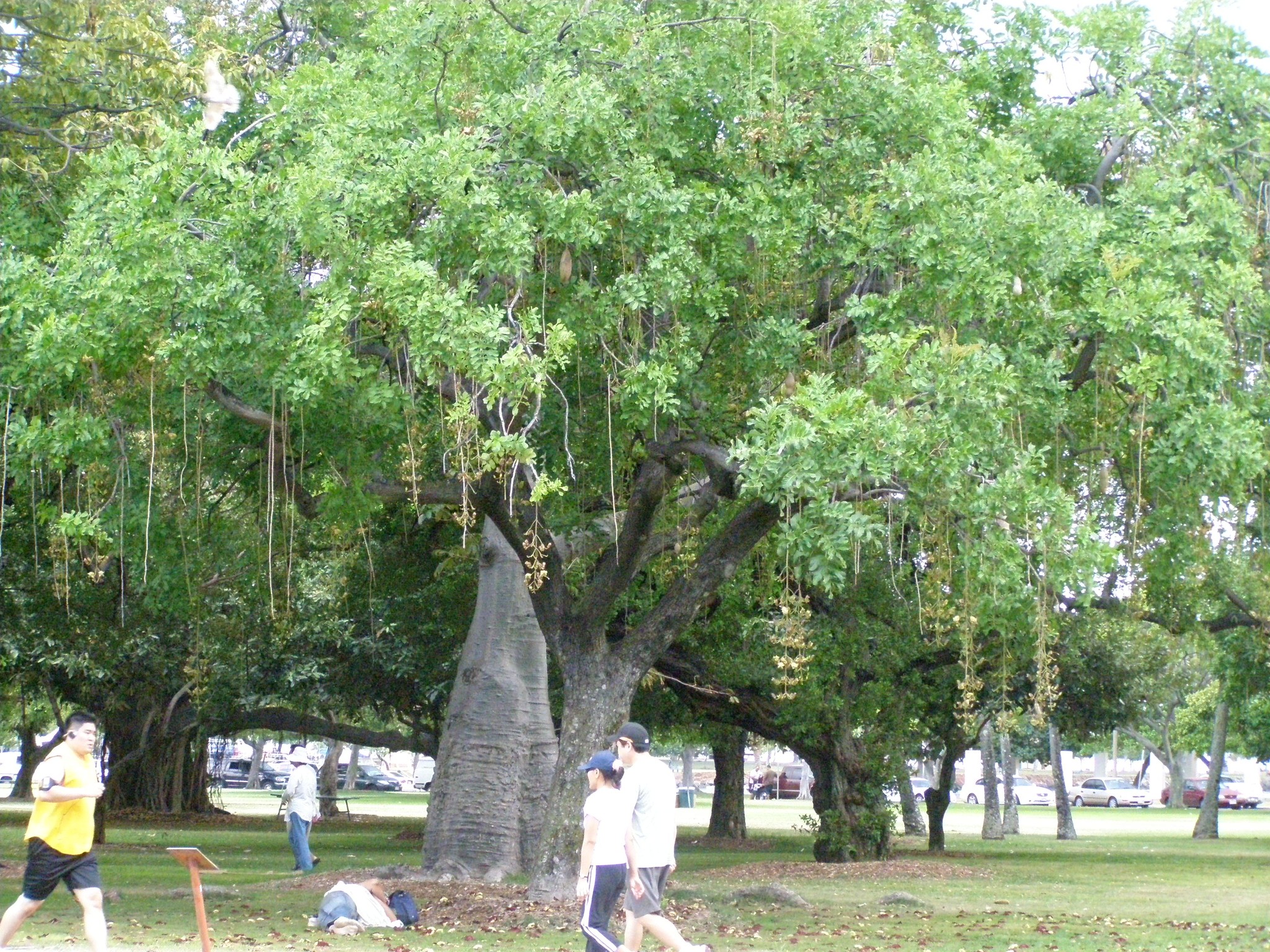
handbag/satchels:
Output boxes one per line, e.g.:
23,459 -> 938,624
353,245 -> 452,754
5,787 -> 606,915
389,889 -> 419,924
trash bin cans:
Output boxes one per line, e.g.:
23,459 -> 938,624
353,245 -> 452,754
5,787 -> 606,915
678,787 -> 695,808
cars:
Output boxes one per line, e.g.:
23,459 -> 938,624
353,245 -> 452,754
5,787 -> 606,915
883,776 -> 932,803
1068,776 -> 1153,809
961,775 -> 1053,806
0,732 -> 437,792
1160,775 -> 1266,810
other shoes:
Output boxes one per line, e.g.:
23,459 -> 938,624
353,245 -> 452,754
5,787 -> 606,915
290,858 -> 320,870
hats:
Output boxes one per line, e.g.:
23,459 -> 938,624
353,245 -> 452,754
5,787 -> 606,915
606,722 -> 650,745
286,745 -> 313,765
577,750 -> 618,771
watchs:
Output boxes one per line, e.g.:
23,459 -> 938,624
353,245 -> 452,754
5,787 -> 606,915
578,873 -> 589,880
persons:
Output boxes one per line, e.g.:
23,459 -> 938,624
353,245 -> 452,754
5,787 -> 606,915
667,760 -> 673,768
280,744 -> 321,870
317,878 -> 402,937
749,779 -> 763,800
571,753 -> 643,952
0,712 -> 112,949
762,765 -> 777,800
604,722 -> 714,952
280,747 -> 316,875
747,766 -> 763,800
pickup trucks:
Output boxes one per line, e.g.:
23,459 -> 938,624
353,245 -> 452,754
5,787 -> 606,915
748,764 -> 814,796
